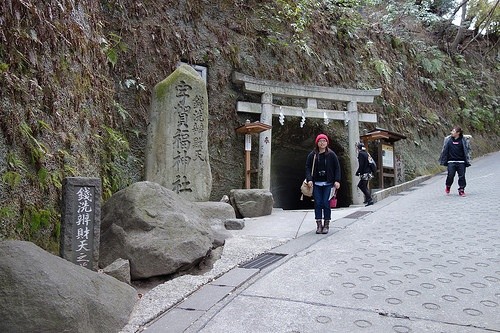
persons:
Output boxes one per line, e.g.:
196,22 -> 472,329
305,134 -> 340,234
438,127 -> 471,197
355,142 -> 377,207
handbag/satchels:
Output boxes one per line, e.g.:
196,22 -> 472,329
368,153 -> 377,172
301,153 -> 317,197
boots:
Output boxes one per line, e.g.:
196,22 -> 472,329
322,219 -> 330,234
315,218 -> 323,234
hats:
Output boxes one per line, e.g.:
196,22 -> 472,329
315,134 -> 329,145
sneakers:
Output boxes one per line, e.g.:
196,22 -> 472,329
446,186 -> 450,194
458,191 -> 466,197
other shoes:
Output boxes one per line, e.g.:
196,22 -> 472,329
365,199 -> 374,206
363,197 -> 368,203
